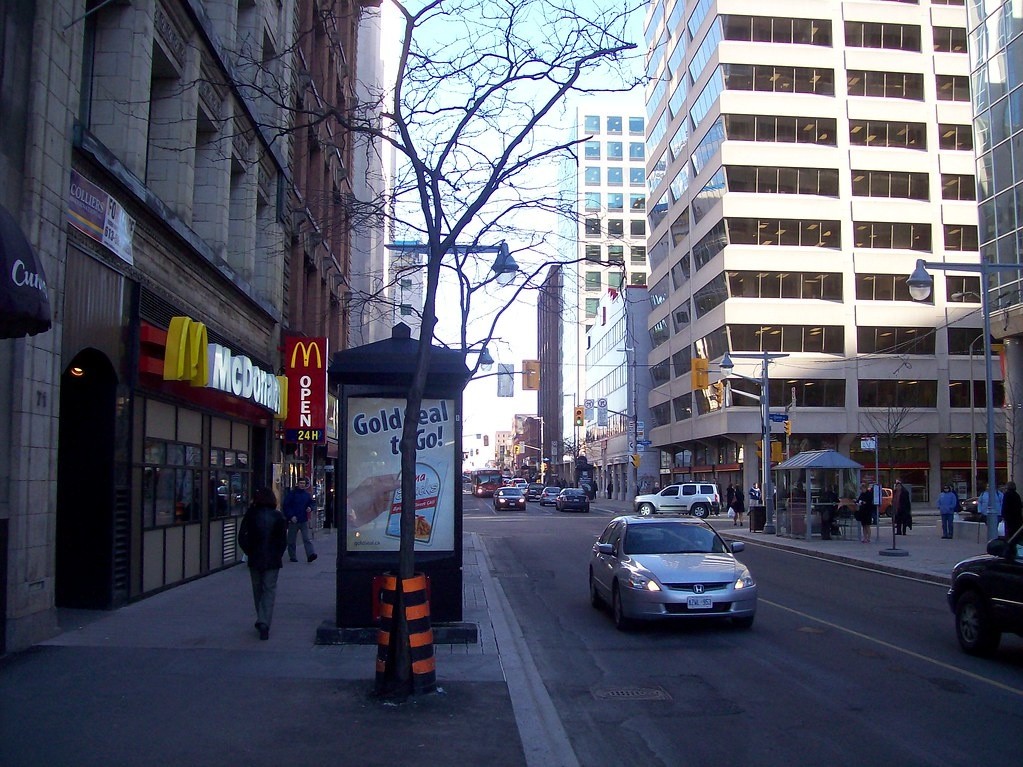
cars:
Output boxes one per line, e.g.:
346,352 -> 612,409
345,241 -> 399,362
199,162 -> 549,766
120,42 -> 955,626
961,497 -> 979,514
588,515 -> 758,628
539,486 -> 561,506
525,482 -> 545,501
555,487 -> 590,513
947,526 -> 1023,657
502,477 -> 529,495
833,488 -> 893,518
493,487 -> 526,511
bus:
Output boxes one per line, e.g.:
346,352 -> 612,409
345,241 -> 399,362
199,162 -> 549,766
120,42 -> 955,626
462,468 -> 502,497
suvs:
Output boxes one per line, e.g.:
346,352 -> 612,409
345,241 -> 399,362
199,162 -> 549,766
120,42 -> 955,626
633,480 -> 721,518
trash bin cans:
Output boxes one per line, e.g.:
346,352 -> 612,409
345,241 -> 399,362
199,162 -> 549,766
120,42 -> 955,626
750,505 -> 766,531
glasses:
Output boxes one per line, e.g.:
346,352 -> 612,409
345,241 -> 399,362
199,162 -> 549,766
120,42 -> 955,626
944,488 -> 949,489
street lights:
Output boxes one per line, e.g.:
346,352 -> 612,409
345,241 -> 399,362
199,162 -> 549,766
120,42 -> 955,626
616,347 -> 642,506
564,393 -> 576,467
719,350 -> 776,534
906,258 -> 1023,545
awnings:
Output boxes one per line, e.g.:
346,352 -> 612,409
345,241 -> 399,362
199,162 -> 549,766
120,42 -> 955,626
673,464 -> 740,473
0,208 -> 53,340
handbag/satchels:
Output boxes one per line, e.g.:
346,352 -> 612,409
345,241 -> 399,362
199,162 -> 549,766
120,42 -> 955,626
997,519 -> 1005,536
728,507 -> 735,519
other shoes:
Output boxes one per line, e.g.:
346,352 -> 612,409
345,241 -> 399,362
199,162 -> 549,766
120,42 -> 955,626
734,523 -> 737,526
290,559 -> 299,563
740,521 -> 742,526
947,536 -> 953,539
904,533 -> 906,536
308,553 -> 317,562
895,533 -> 902,535
260,624 -> 270,640
861,539 -> 871,544
941,536 -> 946,539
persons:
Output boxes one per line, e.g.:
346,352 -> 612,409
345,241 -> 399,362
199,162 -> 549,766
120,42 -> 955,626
999,482 -> 1007,493
593,481 -> 599,499
733,485 -> 745,525
978,484 -> 1004,543
895,477 -> 904,488
855,483 -> 873,543
238,487 -> 287,634
1003,482 -> 1023,545
894,482 -> 912,536
713,478 -> 724,517
936,485 -> 957,539
747,483 -> 761,516
662,484 -> 668,488
553,477 -> 567,485
893,482 -> 894,483
652,481 -> 661,493
726,483 -> 735,513
283,478 -> 318,563
949,484 -> 958,512
817,491 -> 840,541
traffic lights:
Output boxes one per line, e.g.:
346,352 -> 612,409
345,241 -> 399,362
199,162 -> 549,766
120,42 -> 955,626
784,420 -> 792,438
574,408 -> 584,427
513,445 -> 520,454
755,440 -> 762,457
713,381 -> 723,408
630,454 -> 639,468
